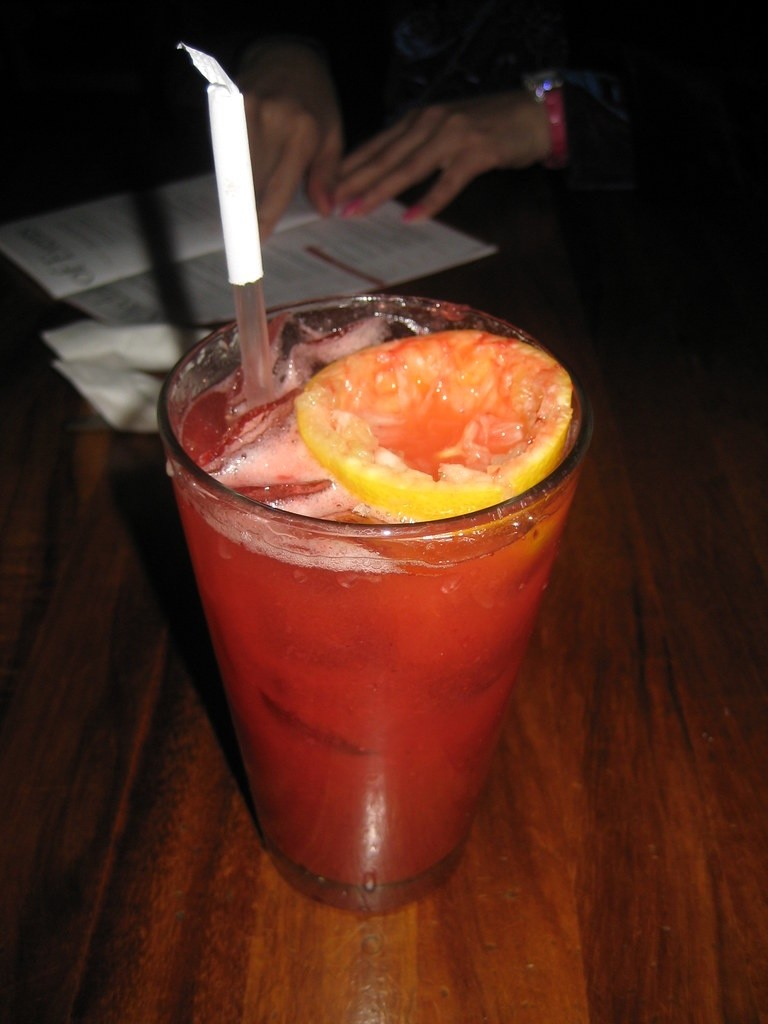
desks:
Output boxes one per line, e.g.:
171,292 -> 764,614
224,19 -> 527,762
0,193 -> 768,1024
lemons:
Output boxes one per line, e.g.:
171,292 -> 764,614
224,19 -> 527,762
296,327 -> 571,520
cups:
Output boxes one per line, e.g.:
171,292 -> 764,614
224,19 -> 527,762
156,294 -> 594,913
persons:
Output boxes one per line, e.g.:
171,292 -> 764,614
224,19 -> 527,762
150,0 -> 637,242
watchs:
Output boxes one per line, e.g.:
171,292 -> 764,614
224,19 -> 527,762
520,66 -> 570,171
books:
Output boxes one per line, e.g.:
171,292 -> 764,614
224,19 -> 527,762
0,165 -> 502,329
